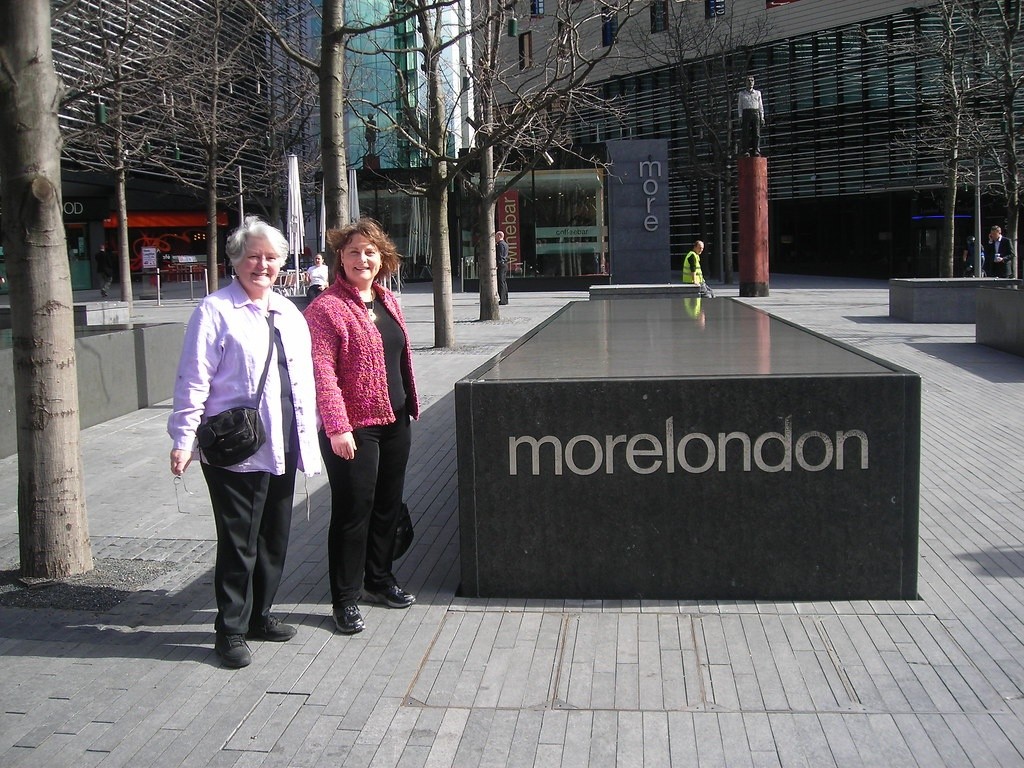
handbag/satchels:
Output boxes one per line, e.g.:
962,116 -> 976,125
389,504 -> 414,570
197,406 -> 266,466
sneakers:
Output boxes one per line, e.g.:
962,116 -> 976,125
333,603 -> 366,632
246,616 -> 298,641
214,632 -> 252,666
363,580 -> 416,607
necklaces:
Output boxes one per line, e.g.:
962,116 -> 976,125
366,287 -> 375,317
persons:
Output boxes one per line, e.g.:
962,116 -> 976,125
495,230 -> 509,306
985,224 -> 1016,279
166,214 -> 325,673
95,244 -> 113,297
962,234 -> 988,278
738,75 -> 765,156
302,216 -> 422,635
681,239 -> 717,299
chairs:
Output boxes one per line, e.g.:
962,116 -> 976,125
230,268 -> 310,297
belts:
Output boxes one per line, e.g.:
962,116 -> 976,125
500,261 -> 507,264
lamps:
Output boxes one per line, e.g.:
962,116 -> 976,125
507,5 -> 517,39
